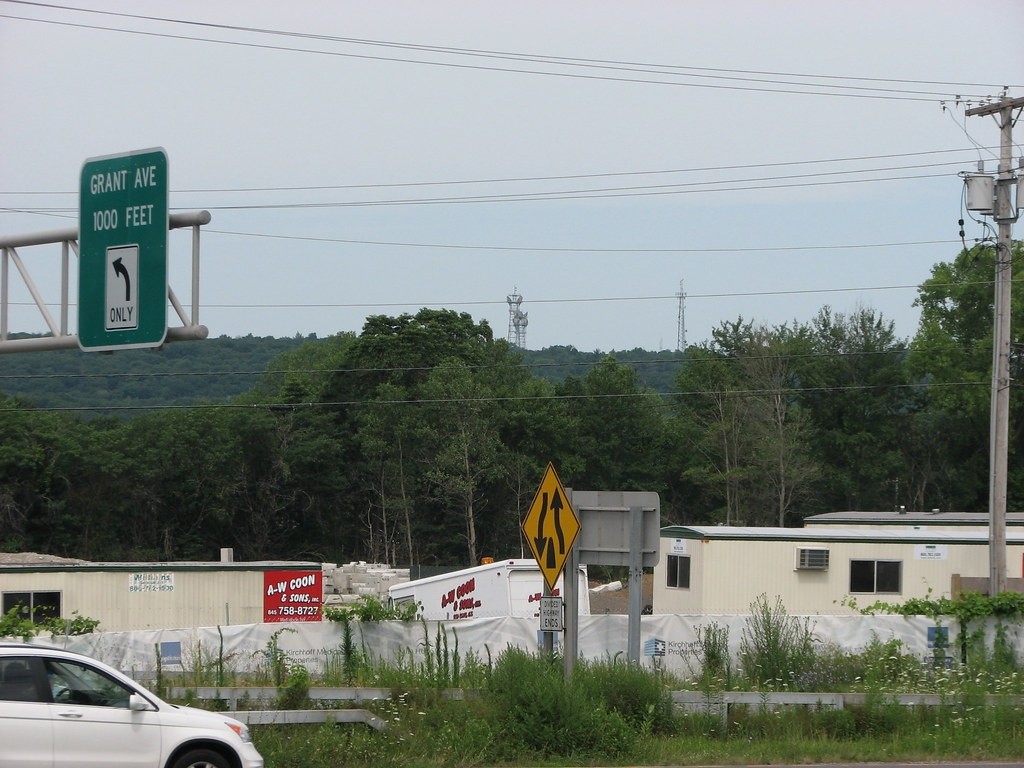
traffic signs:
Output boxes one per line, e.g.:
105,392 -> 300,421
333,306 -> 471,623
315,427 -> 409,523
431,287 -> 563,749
77,144 -> 171,353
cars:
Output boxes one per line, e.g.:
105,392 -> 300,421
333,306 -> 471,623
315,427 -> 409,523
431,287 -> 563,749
0,643 -> 267,768
